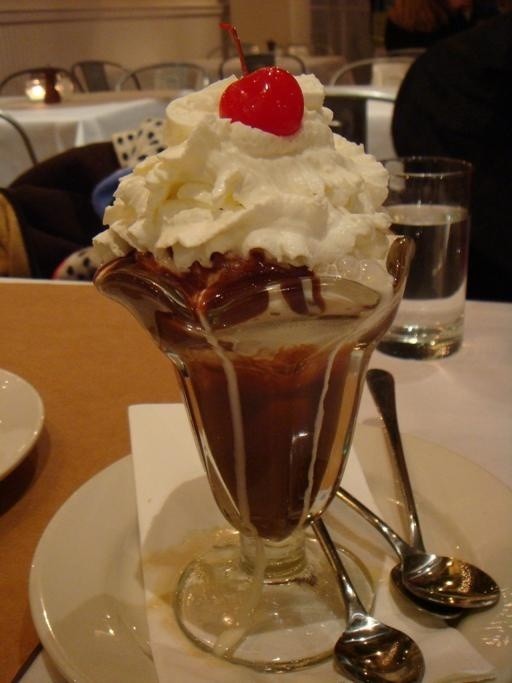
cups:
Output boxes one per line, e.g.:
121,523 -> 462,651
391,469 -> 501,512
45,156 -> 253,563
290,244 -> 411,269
381,153 -> 462,358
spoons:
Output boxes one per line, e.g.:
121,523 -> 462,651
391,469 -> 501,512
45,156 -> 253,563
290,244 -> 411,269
301,507 -> 427,681
362,361 -> 466,624
335,484 -> 498,609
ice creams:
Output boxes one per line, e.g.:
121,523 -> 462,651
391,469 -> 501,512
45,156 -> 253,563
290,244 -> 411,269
96,22 -> 412,543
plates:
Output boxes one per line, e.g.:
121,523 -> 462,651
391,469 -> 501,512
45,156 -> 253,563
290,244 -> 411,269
25,423 -> 510,681
0,358 -> 44,485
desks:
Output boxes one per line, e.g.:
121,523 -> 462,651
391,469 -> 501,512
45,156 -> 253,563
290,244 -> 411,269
1,93 -> 173,190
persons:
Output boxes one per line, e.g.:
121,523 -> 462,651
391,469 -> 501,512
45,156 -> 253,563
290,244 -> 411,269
376,0 -> 512,56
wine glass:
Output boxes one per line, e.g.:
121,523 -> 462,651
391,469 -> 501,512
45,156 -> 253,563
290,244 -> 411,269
98,239 -> 410,672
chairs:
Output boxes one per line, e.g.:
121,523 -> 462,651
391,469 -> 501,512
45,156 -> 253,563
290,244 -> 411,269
2,58 -> 212,92
2,111 -> 38,190
320,93 -> 398,159
212,41 -> 415,87
